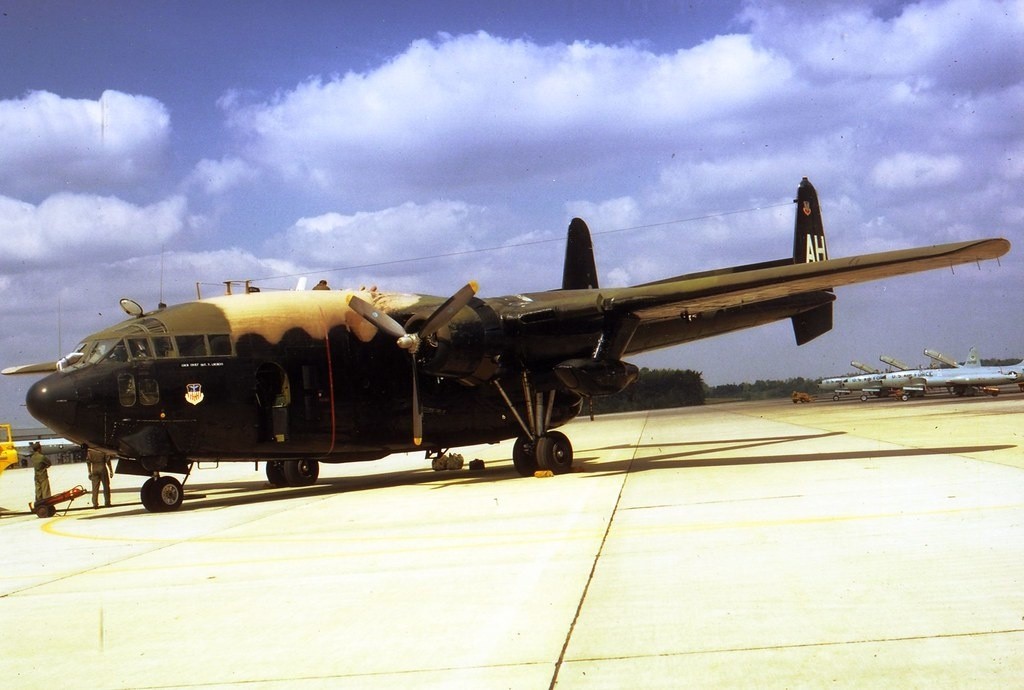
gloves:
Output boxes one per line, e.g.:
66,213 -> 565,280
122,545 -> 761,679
89,474 -> 92,480
110,472 -> 113,478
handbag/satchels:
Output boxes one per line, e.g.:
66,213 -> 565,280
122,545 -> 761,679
432,452 -> 449,471
447,453 -> 464,470
469,459 -> 484,470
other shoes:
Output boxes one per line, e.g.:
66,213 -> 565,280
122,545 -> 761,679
105,504 -> 112,507
94,505 -> 98,509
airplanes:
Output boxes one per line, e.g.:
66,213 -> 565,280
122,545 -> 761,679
0,178 -> 1012,512
817,346 -> 1024,402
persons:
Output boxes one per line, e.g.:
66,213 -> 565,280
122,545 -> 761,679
312,280 -> 330,290
86,448 -> 114,509
30,442 -> 51,501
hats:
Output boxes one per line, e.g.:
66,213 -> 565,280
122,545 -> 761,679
33,443 -> 41,451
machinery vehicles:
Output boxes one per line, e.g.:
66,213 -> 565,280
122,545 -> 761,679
0,424 -> 18,479
792,391 -> 811,404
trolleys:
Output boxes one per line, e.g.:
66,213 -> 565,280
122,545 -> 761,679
28,484 -> 88,518
